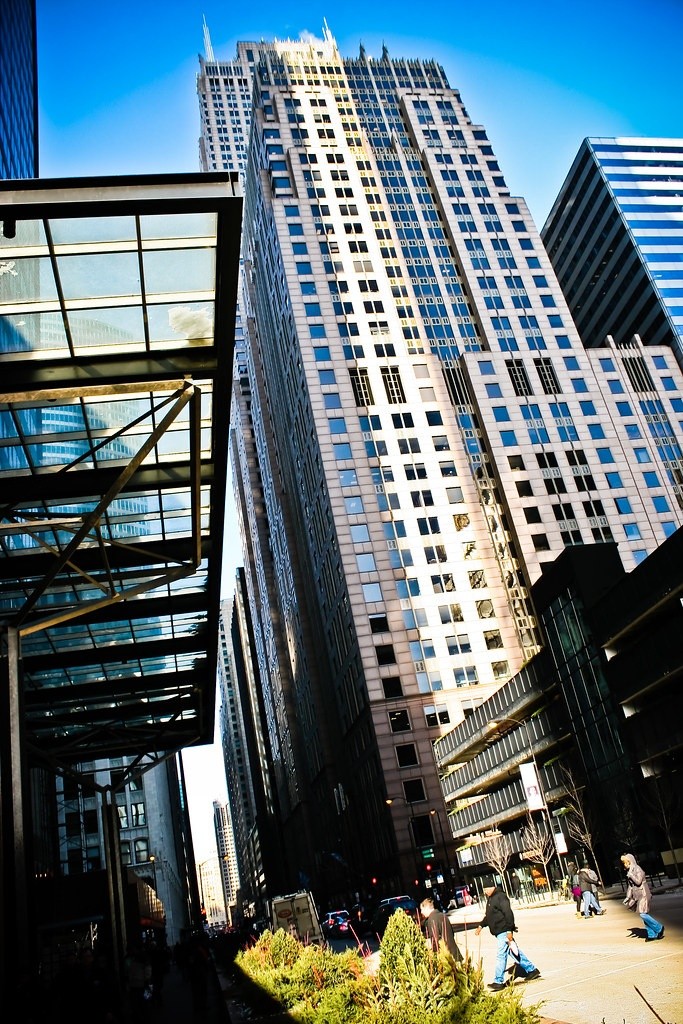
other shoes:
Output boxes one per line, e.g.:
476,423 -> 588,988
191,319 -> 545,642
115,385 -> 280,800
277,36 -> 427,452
658,925 -> 664,938
487,983 -> 504,990
601,909 -> 607,915
645,938 -> 654,942
585,916 -> 593,919
524,968 -> 541,980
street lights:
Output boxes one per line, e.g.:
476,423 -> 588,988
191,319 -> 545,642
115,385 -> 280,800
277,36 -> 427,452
430,808 -> 460,908
487,718 -> 567,882
198,856 -> 230,920
385,798 -> 426,868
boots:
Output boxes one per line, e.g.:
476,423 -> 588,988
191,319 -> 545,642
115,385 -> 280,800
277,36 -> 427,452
577,912 -> 583,919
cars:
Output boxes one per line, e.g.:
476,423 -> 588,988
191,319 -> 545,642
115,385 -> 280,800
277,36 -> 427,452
322,896 -> 426,940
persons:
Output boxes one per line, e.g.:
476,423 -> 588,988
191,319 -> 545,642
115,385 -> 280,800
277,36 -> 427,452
568,860 -> 606,919
620,854 -> 665,943
14,928 -> 244,1024
421,899 -> 464,963
475,878 -> 540,991
448,885 -> 474,909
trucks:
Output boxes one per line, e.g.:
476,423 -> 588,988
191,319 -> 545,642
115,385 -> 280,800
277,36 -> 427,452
265,889 -> 326,950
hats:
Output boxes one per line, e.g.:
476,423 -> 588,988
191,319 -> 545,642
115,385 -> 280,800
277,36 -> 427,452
482,877 -> 495,888
